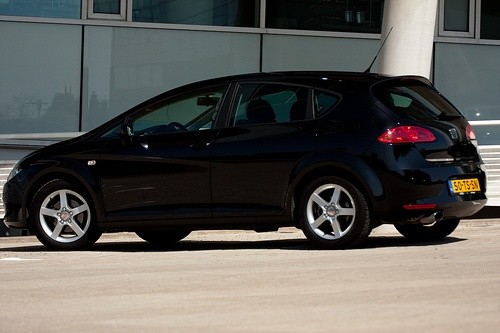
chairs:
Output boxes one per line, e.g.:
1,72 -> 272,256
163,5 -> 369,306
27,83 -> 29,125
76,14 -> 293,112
234,99 -> 307,127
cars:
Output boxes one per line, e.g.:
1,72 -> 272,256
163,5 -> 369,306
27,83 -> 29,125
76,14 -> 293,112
2,27 -> 488,250
